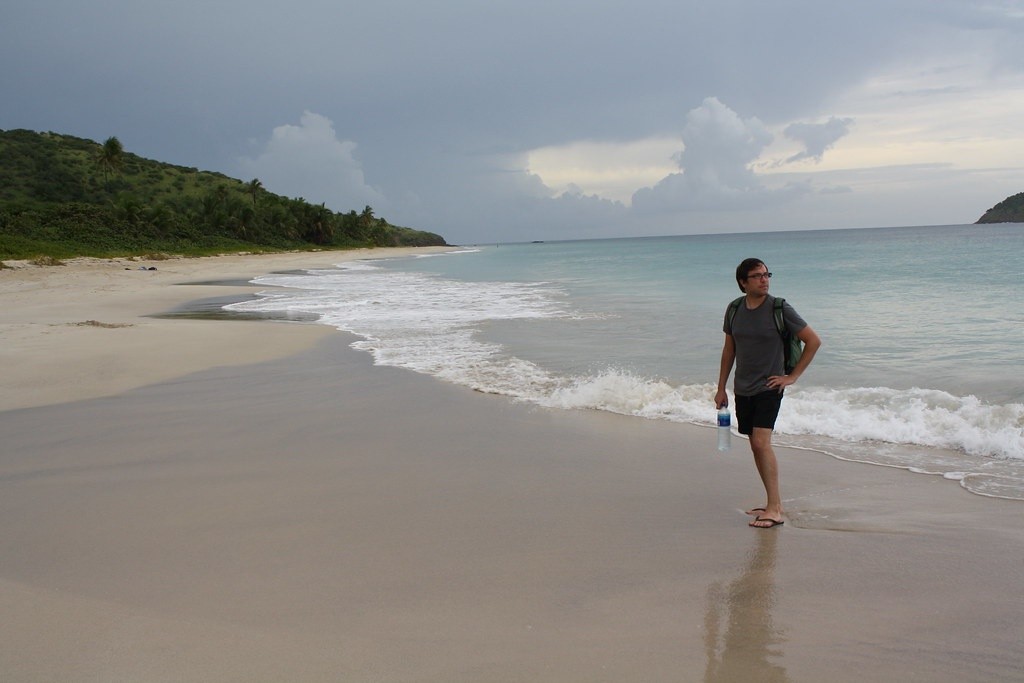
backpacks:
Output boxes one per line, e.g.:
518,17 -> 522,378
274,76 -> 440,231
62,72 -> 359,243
727,297 -> 802,375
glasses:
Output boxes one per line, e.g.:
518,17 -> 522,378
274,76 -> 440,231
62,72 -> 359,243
748,272 -> 772,279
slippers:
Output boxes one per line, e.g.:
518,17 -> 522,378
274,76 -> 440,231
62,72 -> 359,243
749,516 -> 784,528
752,508 -> 766,512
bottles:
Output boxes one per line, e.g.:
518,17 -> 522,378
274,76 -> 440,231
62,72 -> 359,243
718,404 -> 731,452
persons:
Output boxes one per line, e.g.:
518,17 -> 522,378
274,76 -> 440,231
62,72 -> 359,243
714,258 -> 821,528
